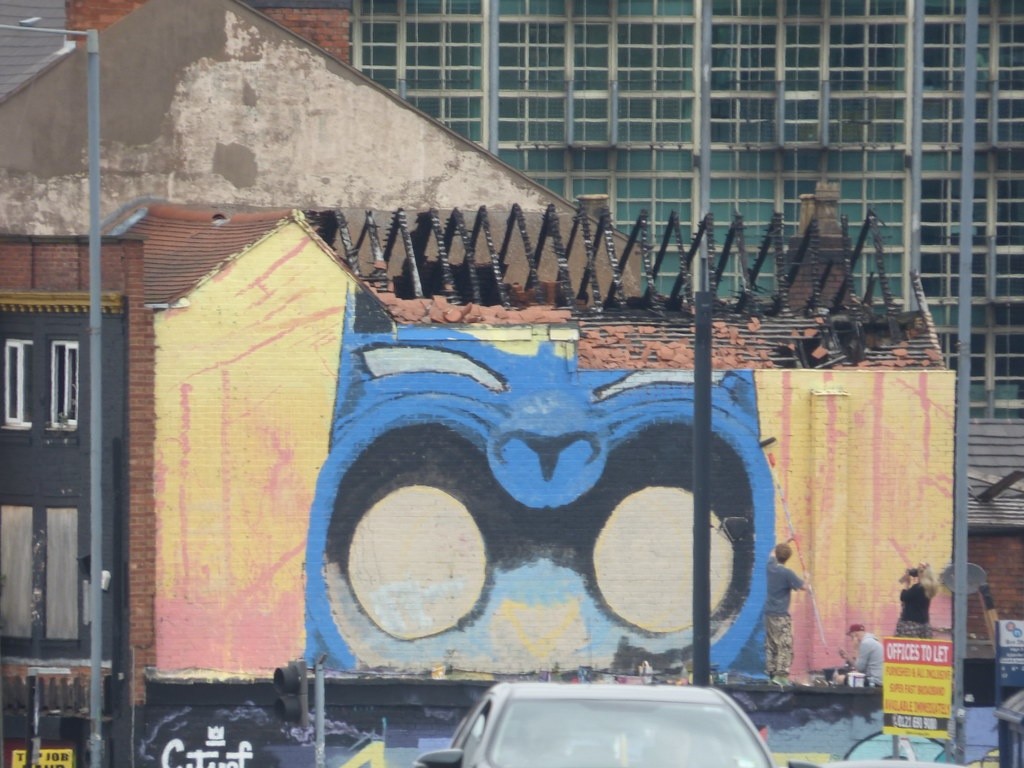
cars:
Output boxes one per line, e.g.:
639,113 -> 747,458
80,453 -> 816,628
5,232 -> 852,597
415,679 -> 788,767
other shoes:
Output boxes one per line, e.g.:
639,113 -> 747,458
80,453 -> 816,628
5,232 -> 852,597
768,675 -> 794,687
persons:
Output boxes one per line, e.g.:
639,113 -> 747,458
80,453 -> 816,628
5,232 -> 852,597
839,624 -> 883,687
766,536 -> 809,686
895,562 -> 938,638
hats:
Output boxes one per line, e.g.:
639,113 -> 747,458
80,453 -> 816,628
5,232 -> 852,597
845,623 -> 865,634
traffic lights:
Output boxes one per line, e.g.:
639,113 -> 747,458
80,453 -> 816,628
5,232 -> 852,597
271,664 -> 304,725
